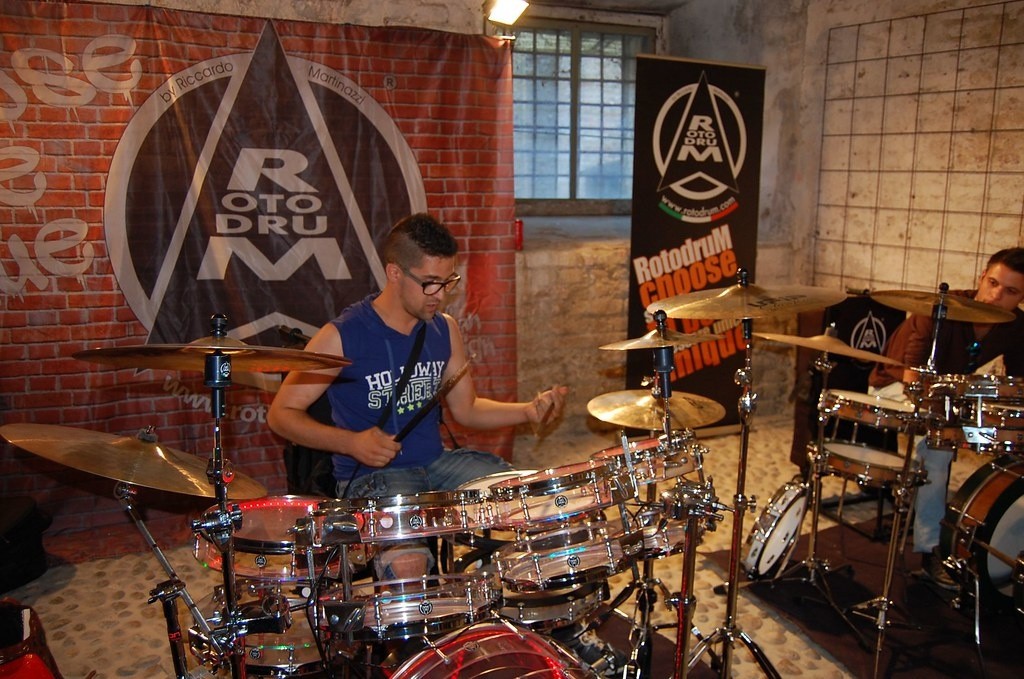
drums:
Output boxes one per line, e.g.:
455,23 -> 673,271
451,470 -> 542,543
939,456 -> 1024,612
187,495 -> 360,679
317,489 -> 491,637
589,431 -> 704,487
489,457 -> 635,531
819,389 -> 929,436
739,481 -> 812,581
904,367 -> 1024,455
389,622 -> 609,679
600,510 -> 703,560
805,440 -> 927,490
491,522 -> 631,630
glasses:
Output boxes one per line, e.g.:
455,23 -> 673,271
398,264 -> 461,295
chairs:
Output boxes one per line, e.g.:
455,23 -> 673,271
279,370 -> 492,676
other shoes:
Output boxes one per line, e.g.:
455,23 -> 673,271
918,552 -> 963,589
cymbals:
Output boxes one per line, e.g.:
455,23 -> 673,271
751,331 -> 904,367
645,283 -> 848,320
587,389 -> 727,431
0,422 -> 268,499
867,289 -> 1016,324
598,331 -> 726,351
70,336 -> 352,372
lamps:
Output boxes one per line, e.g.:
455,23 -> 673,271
483,0 -> 532,41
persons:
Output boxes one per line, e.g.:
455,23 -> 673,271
869,246 -> 1024,589
267,212 -> 568,591
